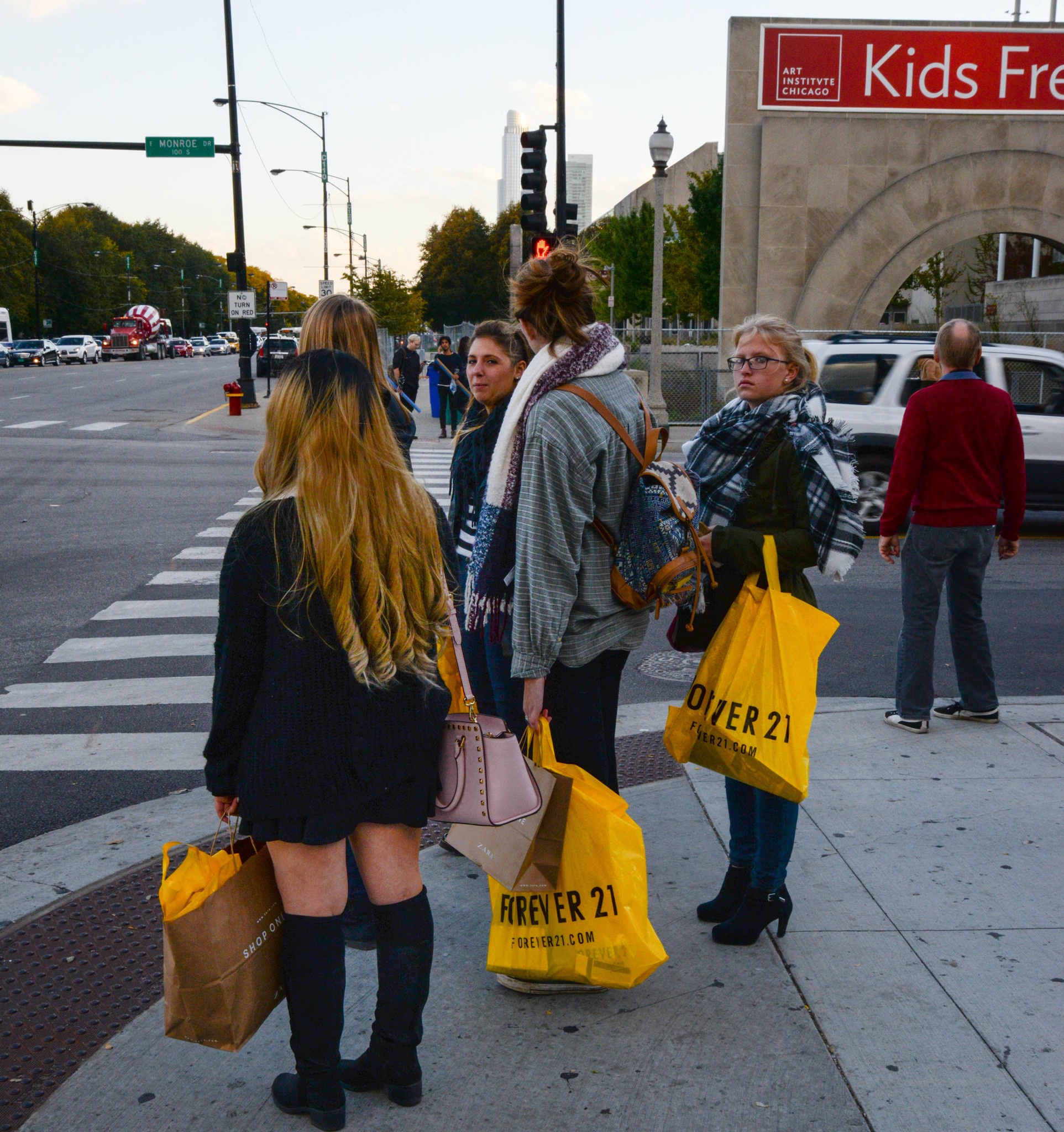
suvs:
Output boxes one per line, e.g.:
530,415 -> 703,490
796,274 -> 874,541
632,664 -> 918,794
55,335 -> 99,363
793,336 -> 1064,533
221,337 -> 240,354
94,334 -> 111,341
255,336 -> 300,377
190,336 -> 208,344
206,335 -> 221,343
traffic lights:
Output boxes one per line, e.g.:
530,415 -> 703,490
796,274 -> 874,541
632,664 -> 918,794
265,318 -> 268,326
521,129 -> 578,261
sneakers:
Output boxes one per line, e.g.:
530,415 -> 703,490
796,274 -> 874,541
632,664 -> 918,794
884,710 -> 930,733
932,699 -> 1000,723
495,971 -> 607,996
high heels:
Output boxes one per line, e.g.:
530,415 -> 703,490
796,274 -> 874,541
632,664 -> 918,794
697,866 -> 751,923
712,883 -> 793,946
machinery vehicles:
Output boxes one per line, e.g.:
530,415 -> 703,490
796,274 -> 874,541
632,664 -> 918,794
101,305 -> 175,362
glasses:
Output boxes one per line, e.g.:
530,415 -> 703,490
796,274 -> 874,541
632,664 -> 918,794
726,355 -> 790,371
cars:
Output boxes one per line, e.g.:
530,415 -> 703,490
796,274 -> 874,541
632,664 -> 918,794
166,340 -> 194,357
0,342 -> 9,368
8,339 -> 59,366
91,340 -> 103,358
210,339 -> 231,355
190,340 -> 211,357
256,336 -> 263,350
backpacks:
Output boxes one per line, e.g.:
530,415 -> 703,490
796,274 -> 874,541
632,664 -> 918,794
552,371 -> 703,612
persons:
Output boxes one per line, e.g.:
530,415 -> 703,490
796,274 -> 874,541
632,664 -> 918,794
475,238 -> 687,998
189,348 -> 481,1132
297,290 -> 417,473
392,333 -> 427,441
432,335 -> 464,438
457,336 -> 471,383
671,308 -> 851,949
879,317 -> 1031,735
432,318 -> 537,738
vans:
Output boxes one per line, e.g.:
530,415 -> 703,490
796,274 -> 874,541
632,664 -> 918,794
0,307 -> 13,350
158,319 -> 172,341
216,331 -> 237,337
248,327 -> 268,337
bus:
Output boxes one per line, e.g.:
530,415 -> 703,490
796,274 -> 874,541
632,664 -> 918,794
278,327 -> 302,337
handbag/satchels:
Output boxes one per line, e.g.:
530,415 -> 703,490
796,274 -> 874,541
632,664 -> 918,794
159,805 -> 289,1054
663,533 -> 840,805
387,348 -> 406,392
486,717 -> 669,990
427,712 -> 543,827
445,715 -> 574,894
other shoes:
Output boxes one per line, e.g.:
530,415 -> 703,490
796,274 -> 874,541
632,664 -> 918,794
450,430 -> 457,438
339,912 -> 378,951
438,429 -> 447,438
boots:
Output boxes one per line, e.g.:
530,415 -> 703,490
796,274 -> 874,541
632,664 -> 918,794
270,911 -> 348,1131
338,883 -> 435,1106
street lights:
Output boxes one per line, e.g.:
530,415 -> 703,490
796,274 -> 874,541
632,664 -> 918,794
642,118 -> 677,428
0,93 -> 368,339
